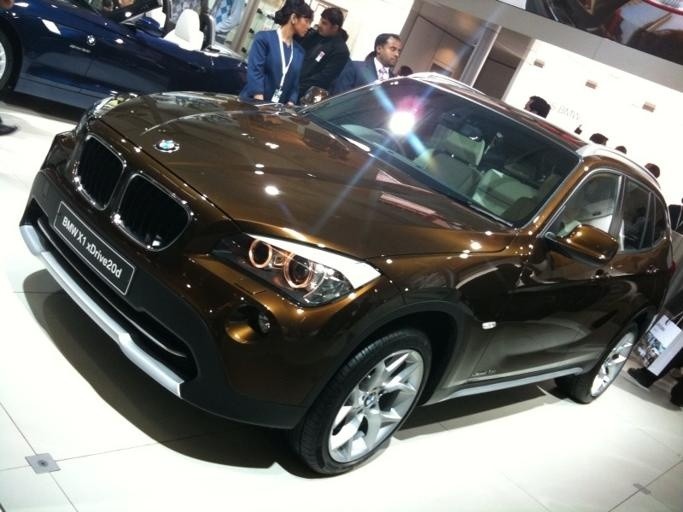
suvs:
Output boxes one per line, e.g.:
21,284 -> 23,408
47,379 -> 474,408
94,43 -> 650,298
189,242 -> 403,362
20,67 -> 677,475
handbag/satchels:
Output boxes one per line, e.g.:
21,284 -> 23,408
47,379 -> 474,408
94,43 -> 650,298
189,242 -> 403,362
635,315 -> 683,375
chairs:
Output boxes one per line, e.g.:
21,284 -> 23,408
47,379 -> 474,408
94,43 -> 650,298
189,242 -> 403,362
412,125 -> 487,197
472,155 -> 551,224
122,4 -> 167,32
164,8 -> 205,52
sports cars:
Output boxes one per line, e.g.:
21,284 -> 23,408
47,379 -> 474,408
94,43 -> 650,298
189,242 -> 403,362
0,0 -> 248,123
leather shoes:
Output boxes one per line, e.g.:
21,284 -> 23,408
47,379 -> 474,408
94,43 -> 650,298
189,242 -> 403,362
0,124 -> 17,135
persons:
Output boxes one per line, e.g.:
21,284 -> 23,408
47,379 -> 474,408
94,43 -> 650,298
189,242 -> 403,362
212,0 -> 244,33
521,94 -> 551,119
363,52 -> 377,61
292,5 -> 350,105
668,196 -> 683,235
0,1 -> 21,136
625,207 -> 649,239
330,31 -> 404,95
391,65 -> 413,75
626,312 -> 682,410
101,0 -> 149,24
615,146 -> 626,155
644,163 -> 660,179
235,0 -> 319,103
588,132 -> 608,147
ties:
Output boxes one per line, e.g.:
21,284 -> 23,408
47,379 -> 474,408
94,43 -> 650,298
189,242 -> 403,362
379,67 -> 385,80
